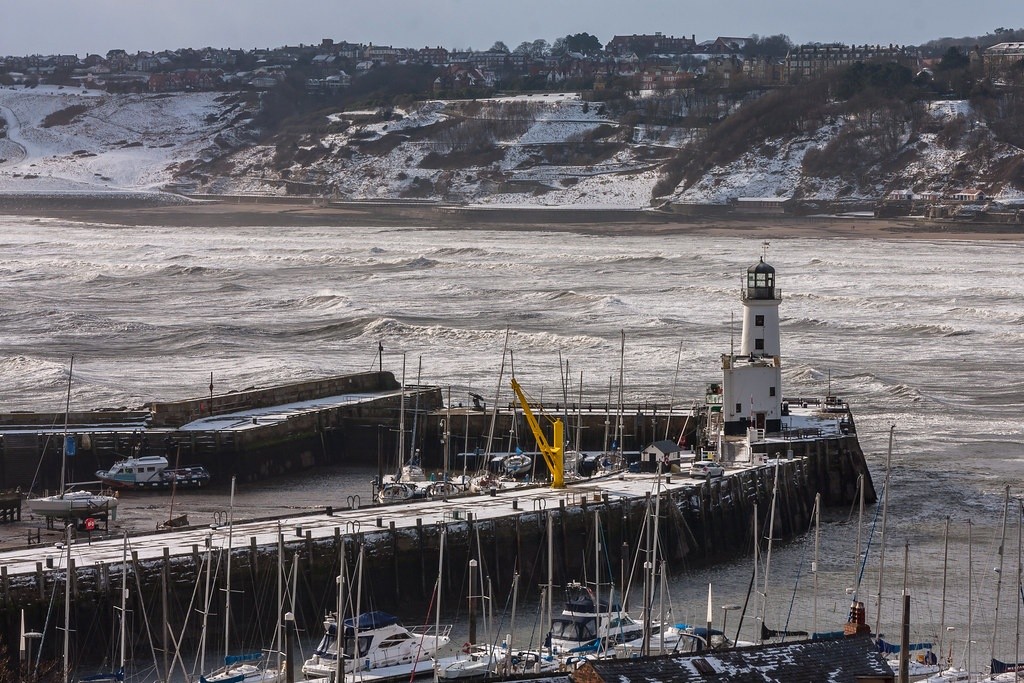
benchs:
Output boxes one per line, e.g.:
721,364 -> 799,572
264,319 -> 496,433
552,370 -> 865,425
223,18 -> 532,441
783,428 -> 822,440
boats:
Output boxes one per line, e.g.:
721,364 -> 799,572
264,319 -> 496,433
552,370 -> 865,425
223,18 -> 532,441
95,455 -> 210,488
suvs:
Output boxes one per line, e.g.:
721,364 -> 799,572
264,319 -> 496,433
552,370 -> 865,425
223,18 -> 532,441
689,461 -> 725,480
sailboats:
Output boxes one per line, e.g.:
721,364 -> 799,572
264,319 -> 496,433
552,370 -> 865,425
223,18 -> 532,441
27,354 -> 119,518
379,326 -> 698,497
1,424 -> 1024,683
